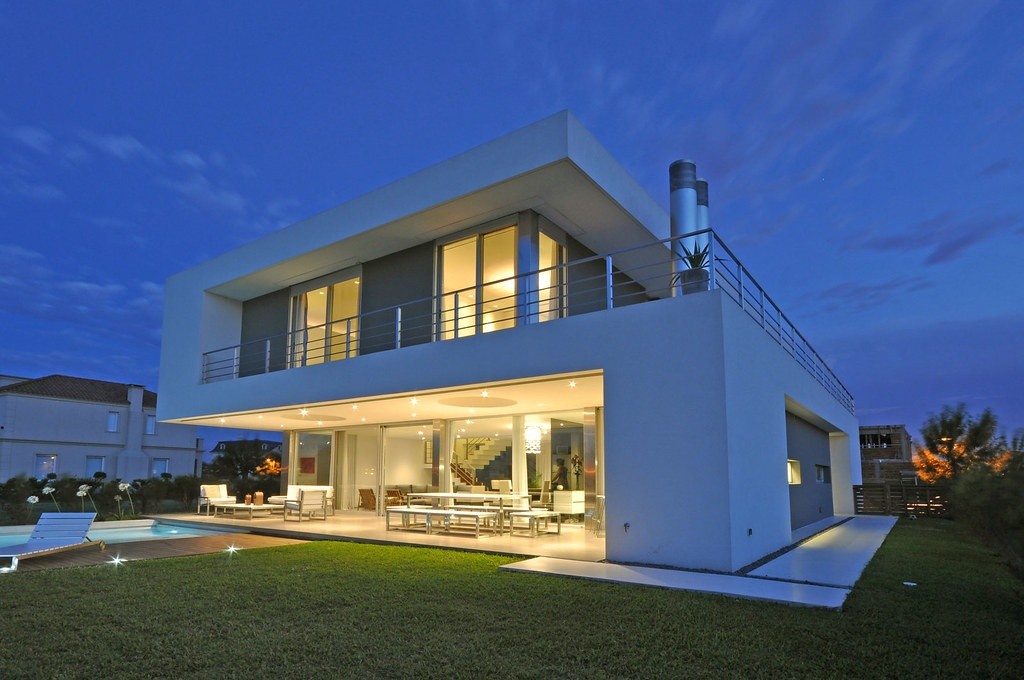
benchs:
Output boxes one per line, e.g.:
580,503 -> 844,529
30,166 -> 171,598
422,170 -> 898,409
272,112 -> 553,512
385,504 -> 433,528
510,511 -> 561,539
428,509 -> 497,539
386,509 -> 447,535
487,507 -> 548,530
444,504 -> 492,528
267,483 -> 336,516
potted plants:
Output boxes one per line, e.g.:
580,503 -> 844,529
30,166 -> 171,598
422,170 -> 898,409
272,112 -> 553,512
668,239 -> 730,295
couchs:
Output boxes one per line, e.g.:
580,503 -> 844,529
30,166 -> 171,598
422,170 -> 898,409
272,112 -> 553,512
379,486 -> 486,506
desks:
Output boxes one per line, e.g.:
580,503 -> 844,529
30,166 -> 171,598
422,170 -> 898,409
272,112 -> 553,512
379,497 -> 398,504
215,503 -> 284,520
407,493 -> 532,536
553,490 -> 585,523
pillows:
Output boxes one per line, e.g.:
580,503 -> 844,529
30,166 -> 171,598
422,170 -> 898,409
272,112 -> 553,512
412,484 -> 427,493
396,485 -> 412,493
384,485 -> 396,495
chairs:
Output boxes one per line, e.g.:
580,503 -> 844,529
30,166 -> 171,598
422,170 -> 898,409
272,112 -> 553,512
532,480 -> 549,507
499,480 -> 512,519
0,513 -> 107,572
387,490 -> 401,506
194,484 -> 236,516
283,487 -> 328,523
357,488 -> 376,511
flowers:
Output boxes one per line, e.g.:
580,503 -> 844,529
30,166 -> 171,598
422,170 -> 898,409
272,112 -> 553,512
570,455 -> 583,473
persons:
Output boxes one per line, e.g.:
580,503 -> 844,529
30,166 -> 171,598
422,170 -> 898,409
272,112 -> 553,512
552,458 -> 568,489
